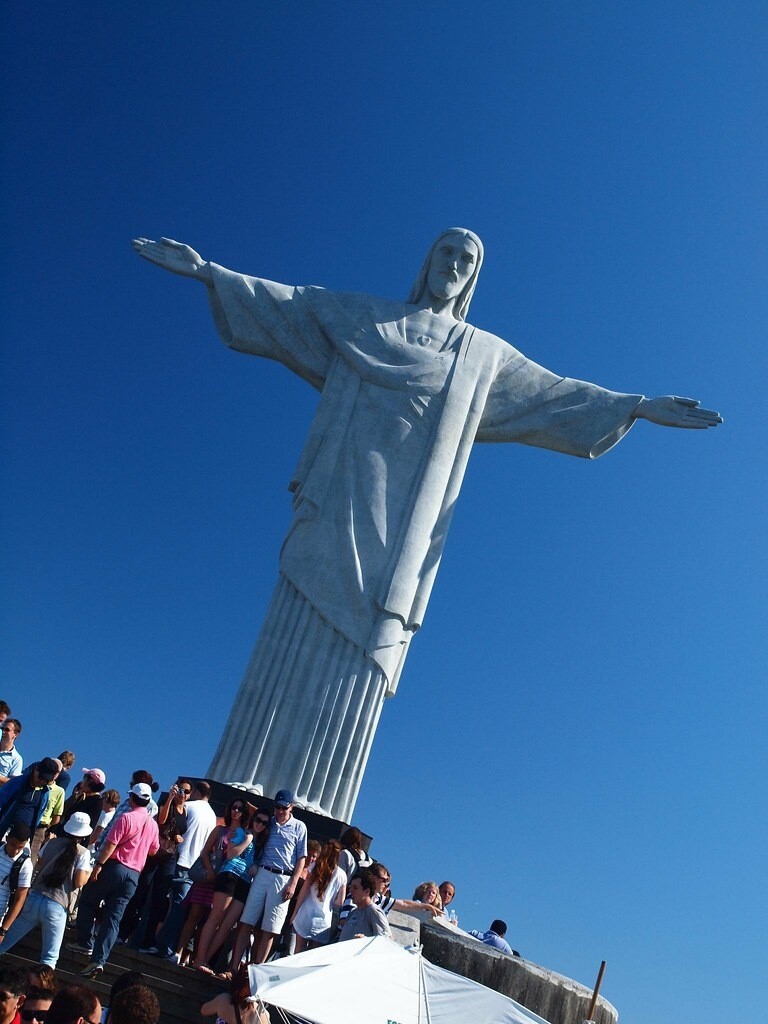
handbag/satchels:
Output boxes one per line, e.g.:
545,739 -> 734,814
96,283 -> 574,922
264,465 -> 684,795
274,924 -> 293,952
159,817 -> 176,840
188,825 -> 223,882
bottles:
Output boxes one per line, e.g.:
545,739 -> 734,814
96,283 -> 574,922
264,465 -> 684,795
450,909 -> 458,922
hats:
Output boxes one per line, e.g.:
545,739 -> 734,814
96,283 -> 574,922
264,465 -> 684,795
82,767 -> 106,785
63,811 -> 93,837
274,790 -> 294,808
38,757 -> 58,781
127,782 -> 151,800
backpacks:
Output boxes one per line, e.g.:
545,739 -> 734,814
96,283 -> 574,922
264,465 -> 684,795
341,847 -> 370,873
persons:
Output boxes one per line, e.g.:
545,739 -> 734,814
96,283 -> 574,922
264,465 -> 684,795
44,986 -> 102,1024
190,808 -> 271,976
116,778 -> 191,955
252,826 -> 513,965
127,224 -> 725,824
101,972 -> 146,1024
158,779 -> 217,956
18,987 -> 55,1024
28,964 -> 58,993
55,789 -> 121,933
0,959 -> 31,1024
163,797 -> 248,965
212,789 -> 309,972
103,984 -> 160,1024
65,782 -> 160,978
1,812 -> 93,972
0,820 -> 33,945
199,962 -> 271,1024
0,699 -> 160,873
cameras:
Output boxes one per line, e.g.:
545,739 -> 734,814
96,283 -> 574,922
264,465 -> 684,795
176,788 -> 185,796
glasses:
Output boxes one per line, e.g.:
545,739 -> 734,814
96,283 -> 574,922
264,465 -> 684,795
385,882 -> 391,888
274,804 -> 292,810
254,816 -> 268,826
38,772 -> 49,782
83,773 -> 92,780
374,874 -> 388,883
231,805 -> 245,813
73,1015 -> 102,1024
184,789 -> 192,794
21,1010 -> 48,1022
0,992 -> 21,1001
129,781 -> 136,788
